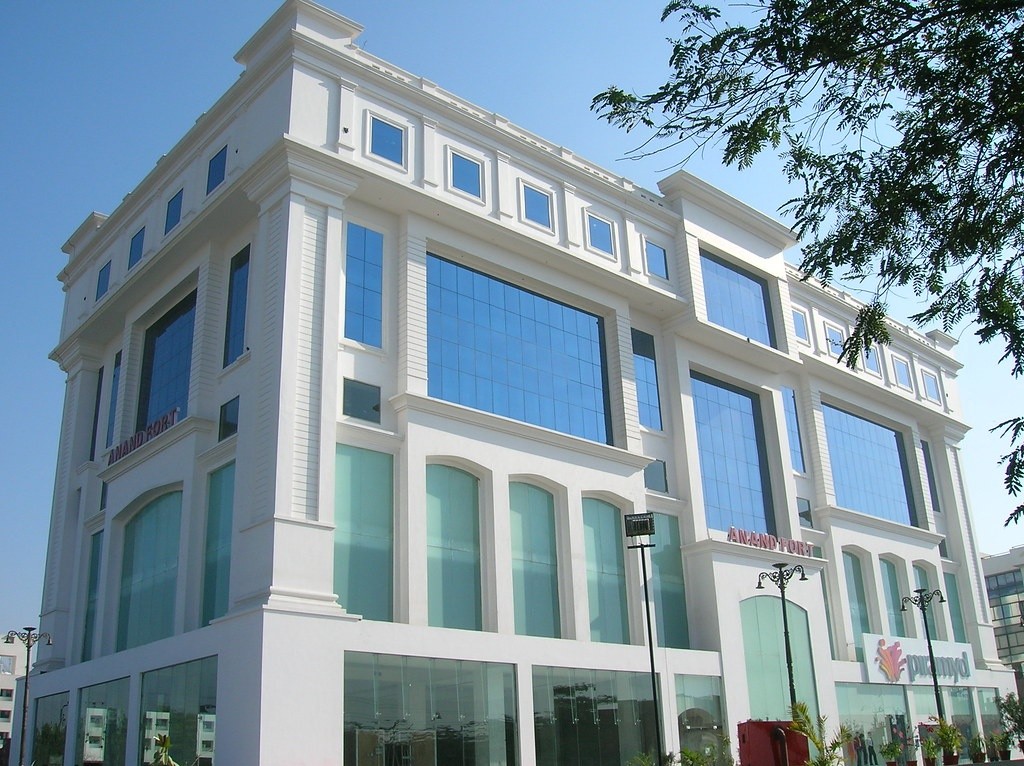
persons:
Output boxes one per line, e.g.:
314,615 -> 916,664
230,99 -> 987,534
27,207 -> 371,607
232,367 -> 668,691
847,732 -> 879,766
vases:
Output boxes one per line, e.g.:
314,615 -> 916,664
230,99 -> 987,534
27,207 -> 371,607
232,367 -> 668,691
905,761 -> 917,766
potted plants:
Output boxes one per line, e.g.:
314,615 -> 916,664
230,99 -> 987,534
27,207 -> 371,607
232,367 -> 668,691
992,692 -> 1024,754
935,718 -> 966,766
879,741 -> 902,766
994,734 -> 1014,761
921,735 -> 941,766
966,734 -> 986,764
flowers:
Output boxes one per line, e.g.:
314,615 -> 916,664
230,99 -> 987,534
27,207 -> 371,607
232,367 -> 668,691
893,721 -> 934,757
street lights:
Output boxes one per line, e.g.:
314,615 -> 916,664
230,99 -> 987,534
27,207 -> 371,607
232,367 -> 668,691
622,512 -> 665,766
756,561 -> 809,722
899,587 -> 950,732
5,626 -> 56,766
